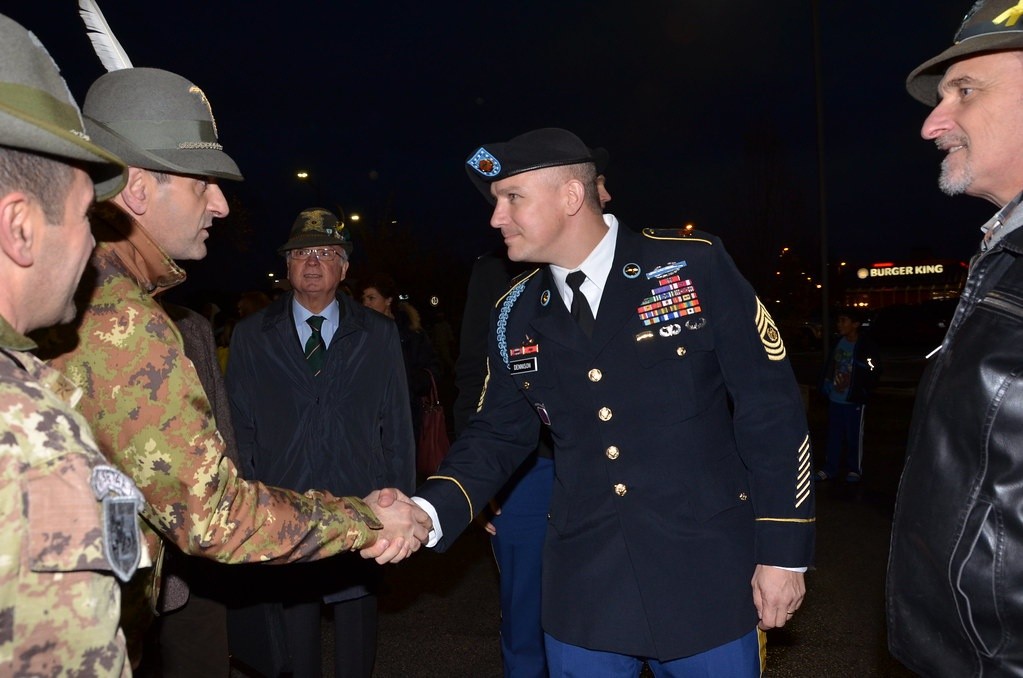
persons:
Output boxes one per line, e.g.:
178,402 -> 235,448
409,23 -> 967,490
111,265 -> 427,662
0,15 -> 874,678
883,0 -> 1022,677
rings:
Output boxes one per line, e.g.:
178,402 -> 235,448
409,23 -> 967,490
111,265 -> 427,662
787,610 -> 796,615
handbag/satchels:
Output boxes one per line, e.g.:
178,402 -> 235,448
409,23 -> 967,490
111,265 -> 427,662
417,368 -> 450,476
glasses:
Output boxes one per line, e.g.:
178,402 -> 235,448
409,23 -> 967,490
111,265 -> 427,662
287,248 -> 342,261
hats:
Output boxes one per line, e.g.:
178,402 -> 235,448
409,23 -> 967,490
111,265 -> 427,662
0,13 -> 129,203
906,0 -> 1023,108
465,128 -> 594,183
277,207 -> 352,257
78,0 -> 244,181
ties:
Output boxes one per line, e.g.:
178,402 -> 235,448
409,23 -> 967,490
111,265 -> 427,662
305,316 -> 327,383
566,270 -> 595,345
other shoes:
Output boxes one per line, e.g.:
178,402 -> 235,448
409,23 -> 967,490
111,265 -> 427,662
815,471 -> 832,481
847,472 -> 863,482
